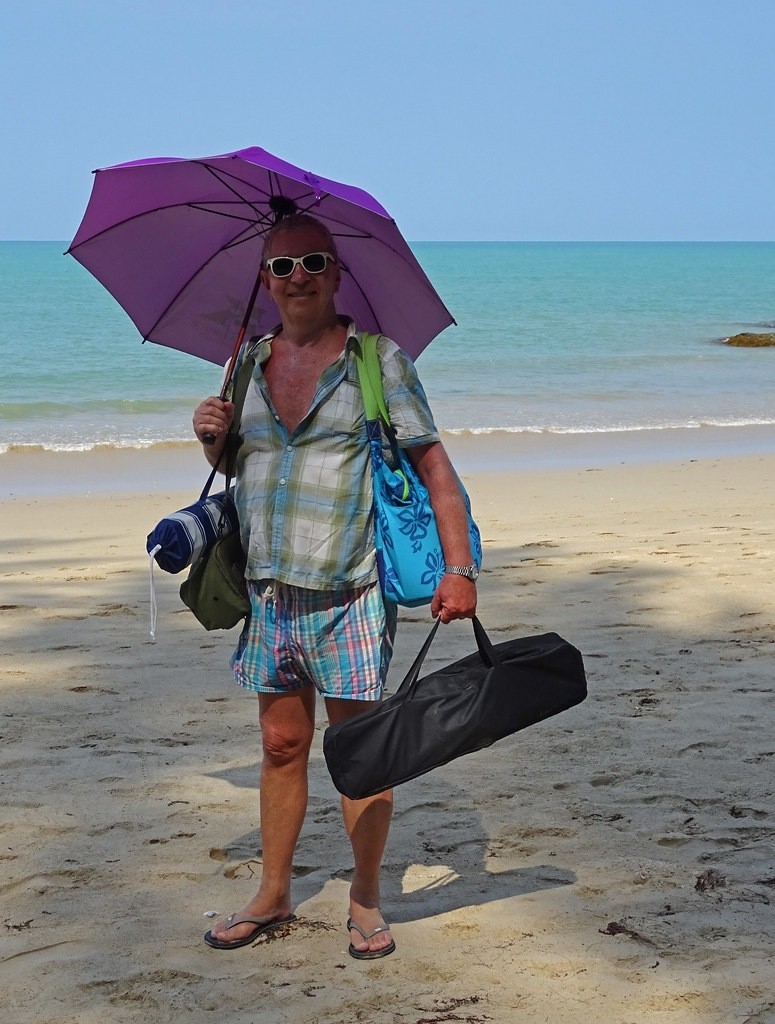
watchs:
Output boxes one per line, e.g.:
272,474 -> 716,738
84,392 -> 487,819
445,564 -> 479,581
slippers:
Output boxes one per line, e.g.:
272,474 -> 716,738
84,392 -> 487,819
204,913 -> 297,950
347,917 -> 396,960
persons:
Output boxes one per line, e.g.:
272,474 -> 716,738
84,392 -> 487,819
193,213 -> 477,960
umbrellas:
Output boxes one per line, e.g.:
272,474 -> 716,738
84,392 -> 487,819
63,145 -> 457,445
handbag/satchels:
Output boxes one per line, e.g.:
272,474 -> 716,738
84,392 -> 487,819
323,613 -> 588,800
357,331 -> 483,608
146,484 -> 252,641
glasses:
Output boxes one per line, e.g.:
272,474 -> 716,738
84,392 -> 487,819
266,252 -> 335,278
